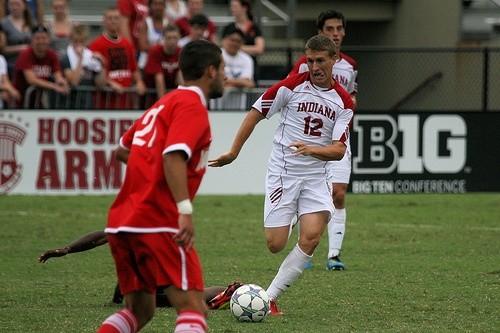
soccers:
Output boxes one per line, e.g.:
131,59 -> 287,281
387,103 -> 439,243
229,283 -> 270,324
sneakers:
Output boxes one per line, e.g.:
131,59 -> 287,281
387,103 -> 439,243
206,283 -> 243,309
304,262 -> 310,270
326,256 -> 344,270
269,300 -> 283,316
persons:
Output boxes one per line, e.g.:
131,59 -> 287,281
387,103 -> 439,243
0,30 -> 20,110
207,35 -> 355,315
283,10 -> 359,270
174,0 -> 215,45
116,0 -> 153,62
0,0 -> 35,80
48,26 -> 104,109
177,15 -> 211,49
88,6 -> 145,110
165,0 -> 188,20
209,29 -> 256,111
38,232 -> 243,310
137,0 -> 174,71
6,26 -> 70,109
219,0 -> 265,87
42,0 -> 78,51
96,40 -> 228,333
143,25 -> 185,101
